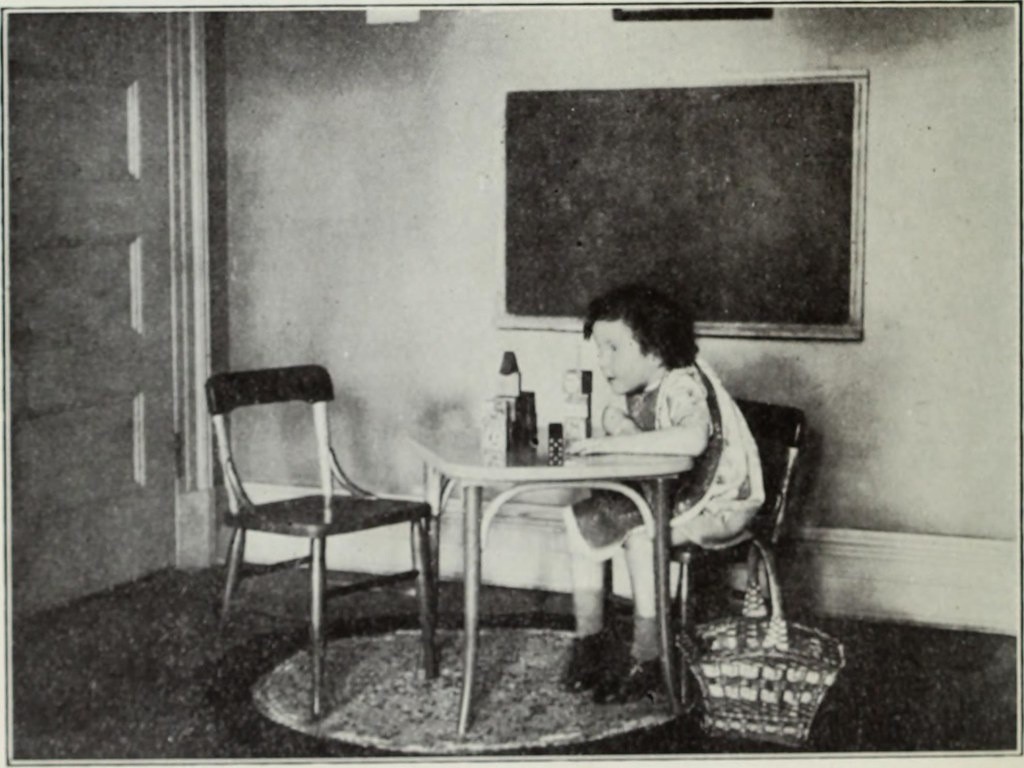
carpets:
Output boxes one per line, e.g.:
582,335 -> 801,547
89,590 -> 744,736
252,628 -> 679,754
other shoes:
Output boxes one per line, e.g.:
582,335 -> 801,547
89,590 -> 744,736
590,654 -> 664,704
557,629 -> 627,693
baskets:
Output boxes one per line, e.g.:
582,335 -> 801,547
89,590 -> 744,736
675,538 -> 846,746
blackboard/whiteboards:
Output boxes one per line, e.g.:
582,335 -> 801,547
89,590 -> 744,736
500,64 -> 871,342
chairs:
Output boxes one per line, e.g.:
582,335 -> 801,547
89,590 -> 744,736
666,397 -> 807,701
204,363 -> 436,720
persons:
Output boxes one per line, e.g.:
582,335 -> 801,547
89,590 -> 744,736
560,284 -> 764,705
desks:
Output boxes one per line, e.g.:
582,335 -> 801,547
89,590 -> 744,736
415,418 -> 693,732
